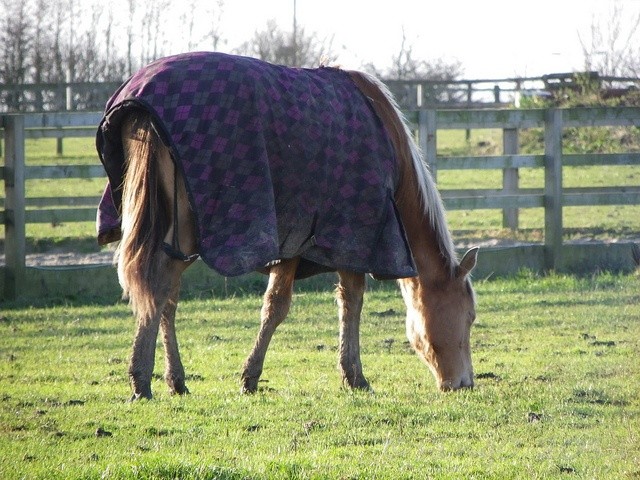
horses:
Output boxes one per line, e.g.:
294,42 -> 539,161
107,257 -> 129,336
111,52 -> 479,402
